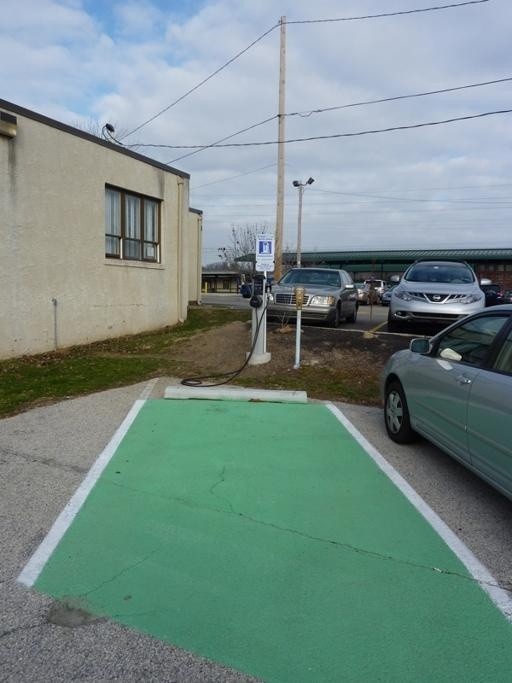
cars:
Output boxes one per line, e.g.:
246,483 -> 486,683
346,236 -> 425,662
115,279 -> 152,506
378,304 -> 511,499
265,266 -> 361,328
352,276 -> 512,308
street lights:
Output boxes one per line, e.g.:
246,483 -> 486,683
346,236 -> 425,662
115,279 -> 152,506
292,176 -> 314,268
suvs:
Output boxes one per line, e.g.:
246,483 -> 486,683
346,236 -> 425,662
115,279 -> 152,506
388,257 -> 490,332
